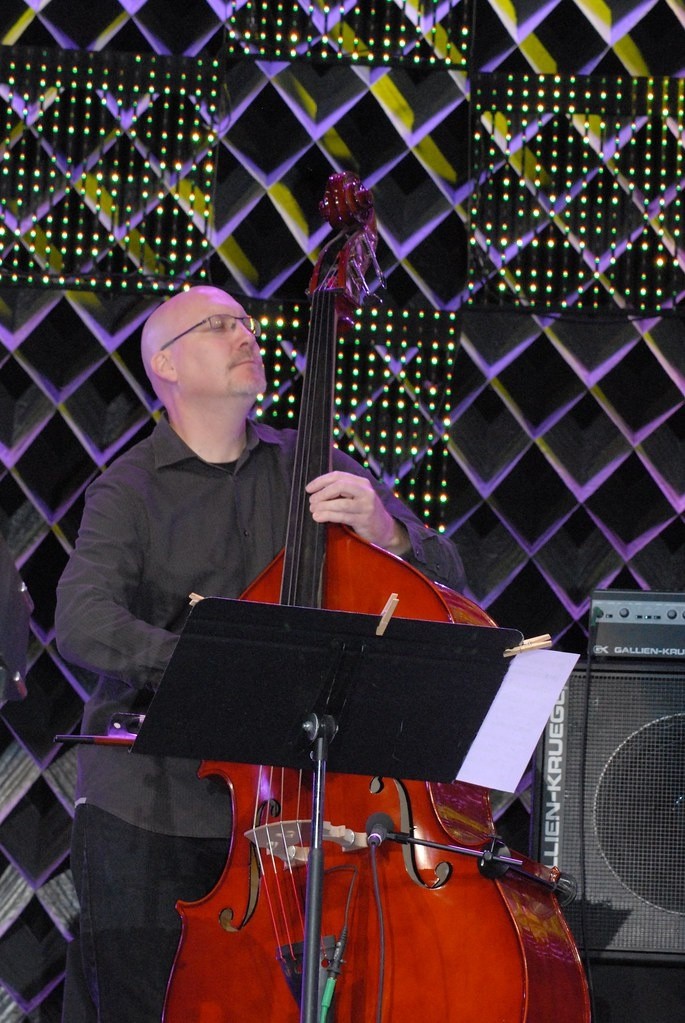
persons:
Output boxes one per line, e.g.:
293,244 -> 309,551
54,285 -> 467,1023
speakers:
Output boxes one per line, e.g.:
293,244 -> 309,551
528,658 -> 684,969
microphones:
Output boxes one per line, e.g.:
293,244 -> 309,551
365,810 -> 395,848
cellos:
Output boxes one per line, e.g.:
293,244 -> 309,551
157,170 -> 594,1023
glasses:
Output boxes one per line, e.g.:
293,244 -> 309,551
160,313 -> 261,353
